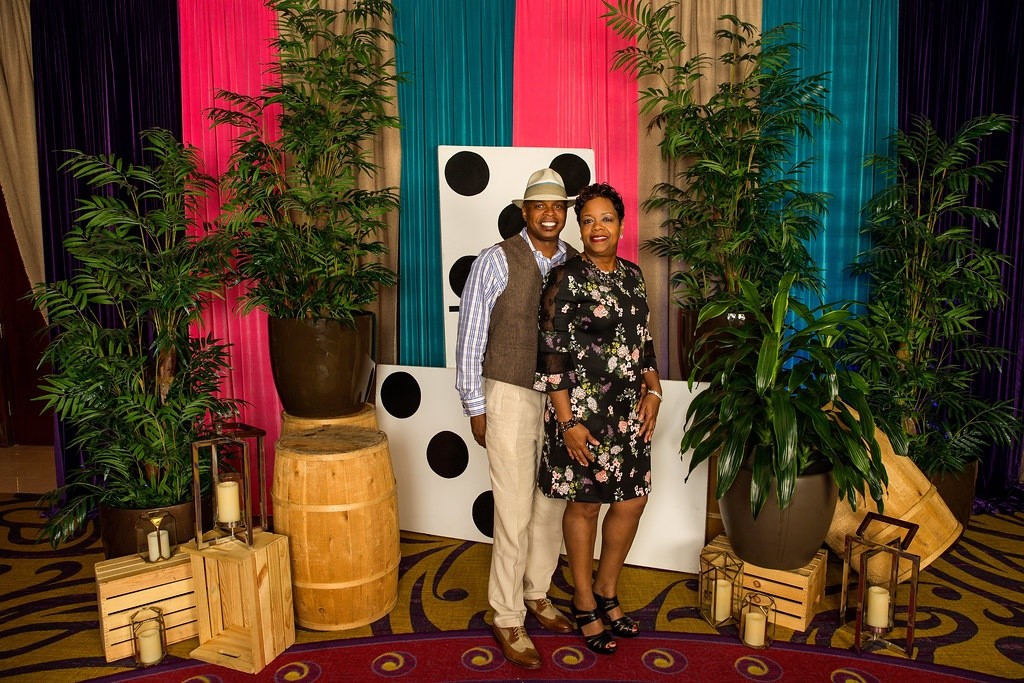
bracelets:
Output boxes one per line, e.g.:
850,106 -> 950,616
646,389 -> 663,402
559,416 -> 578,433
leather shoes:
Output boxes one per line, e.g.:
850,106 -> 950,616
525,598 -> 573,633
493,622 -> 542,669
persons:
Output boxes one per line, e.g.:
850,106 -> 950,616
455,168 -> 646,669
538,182 -> 662,654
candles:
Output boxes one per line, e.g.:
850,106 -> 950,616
711,579 -> 731,621
147,530 -> 170,561
216,482 -> 241,523
138,630 -> 162,663
868,586 -> 889,628
745,613 -> 766,646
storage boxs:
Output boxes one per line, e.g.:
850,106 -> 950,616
698,530 -> 828,632
180,526 -> 296,675
94,530 -> 210,664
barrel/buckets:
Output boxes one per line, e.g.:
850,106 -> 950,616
273,428 -> 401,631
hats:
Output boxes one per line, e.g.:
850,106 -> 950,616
512,168 -> 576,208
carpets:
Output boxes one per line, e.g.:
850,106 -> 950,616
76,627 -> 1017,683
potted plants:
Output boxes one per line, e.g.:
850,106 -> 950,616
596,0 -> 833,382
829,106 -> 1024,558
21,127 -> 254,563
679,271 -> 910,568
211,0 -> 417,420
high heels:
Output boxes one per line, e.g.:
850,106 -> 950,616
593,592 -> 640,638
570,595 -> 616,653
